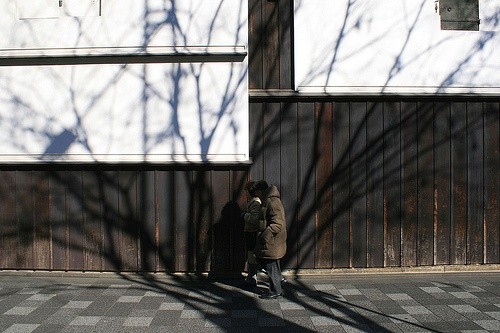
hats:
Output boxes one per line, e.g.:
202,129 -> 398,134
250,179 -> 268,192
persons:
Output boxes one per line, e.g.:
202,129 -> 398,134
241,181 -> 267,291
251,180 -> 287,298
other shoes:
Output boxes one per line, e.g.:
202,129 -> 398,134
280,277 -> 286,287
261,292 -> 283,299
